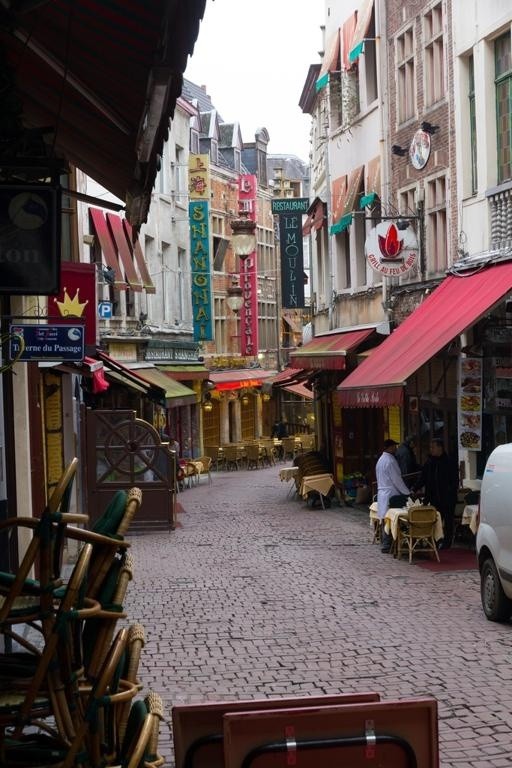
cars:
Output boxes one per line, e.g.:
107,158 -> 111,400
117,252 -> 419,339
476,442 -> 512,620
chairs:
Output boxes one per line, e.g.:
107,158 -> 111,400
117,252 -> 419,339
370,486 -> 483,564
203,434 -> 315,472
177,457 -> 212,492
1,455 -> 170,768
279,450 -> 341,511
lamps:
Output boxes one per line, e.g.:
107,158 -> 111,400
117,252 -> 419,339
93,260 -> 116,284
241,396 -> 249,405
225,280 -> 245,314
204,400 -> 212,412
228,208 -> 256,261
262,392 -> 271,402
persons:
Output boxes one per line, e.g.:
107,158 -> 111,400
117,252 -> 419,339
409,437 -> 459,550
169,435 -> 180,459
270,420 -> 288,462
392,434 -> 424,498
376,438 -> 414,553
154,437 -> 183,492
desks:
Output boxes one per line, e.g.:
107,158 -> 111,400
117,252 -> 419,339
172,692 -> 442,767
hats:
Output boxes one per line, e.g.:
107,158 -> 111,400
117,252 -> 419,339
383,439 -> 400,447
